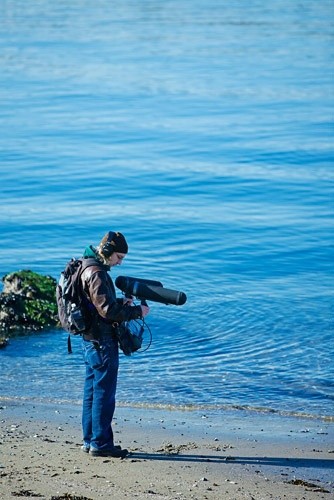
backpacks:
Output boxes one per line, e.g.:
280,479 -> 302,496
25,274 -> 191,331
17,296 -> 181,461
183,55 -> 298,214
55,256 -> 102,354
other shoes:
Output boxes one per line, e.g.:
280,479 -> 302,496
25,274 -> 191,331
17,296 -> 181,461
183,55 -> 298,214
88,446 -> 129,458
83,443 -> 122,454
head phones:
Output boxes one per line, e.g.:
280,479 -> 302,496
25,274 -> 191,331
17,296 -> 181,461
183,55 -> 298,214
102,231 -> 115,258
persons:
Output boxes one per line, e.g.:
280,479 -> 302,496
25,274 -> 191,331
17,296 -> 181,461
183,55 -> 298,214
56,231 -> 149,458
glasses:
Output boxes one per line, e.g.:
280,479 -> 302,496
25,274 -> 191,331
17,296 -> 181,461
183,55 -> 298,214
114,252 -> 122,260
